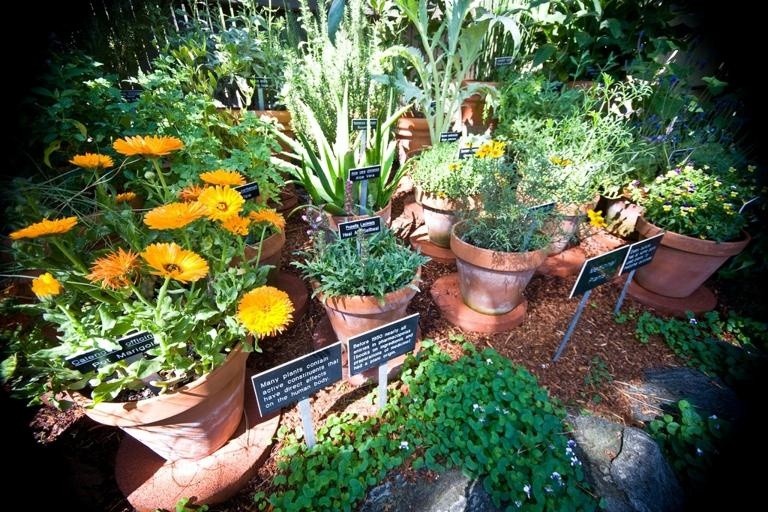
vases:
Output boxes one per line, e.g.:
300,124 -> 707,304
71,333 -> 254,462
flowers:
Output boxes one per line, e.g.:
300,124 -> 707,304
1,134 -> 295,412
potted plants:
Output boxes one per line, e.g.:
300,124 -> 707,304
34,1 -> 763,352
